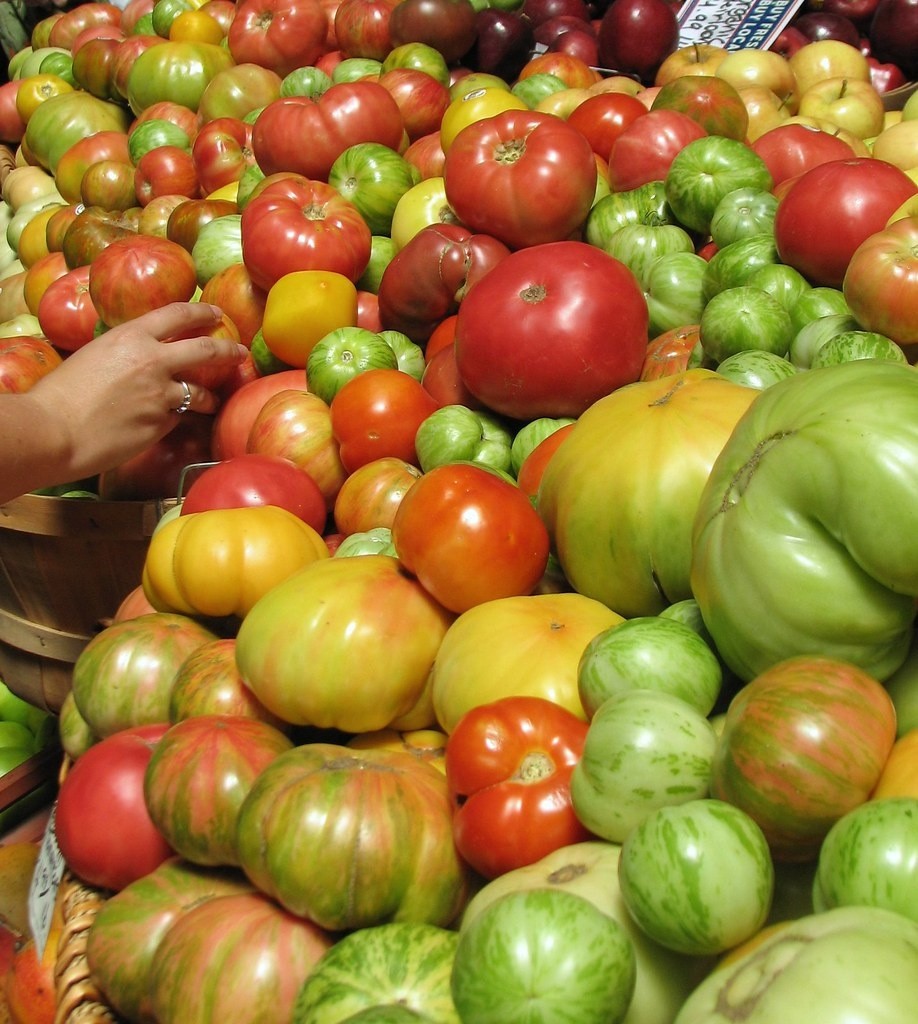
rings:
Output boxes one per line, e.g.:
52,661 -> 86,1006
176,379 -> 191,414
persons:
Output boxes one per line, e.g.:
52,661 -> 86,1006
0,0 -> 96,60
0,302 -> 247,510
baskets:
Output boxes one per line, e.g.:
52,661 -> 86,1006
53,869 -> 113,1024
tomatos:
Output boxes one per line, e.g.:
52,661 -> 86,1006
1,1 -> 916,1024
